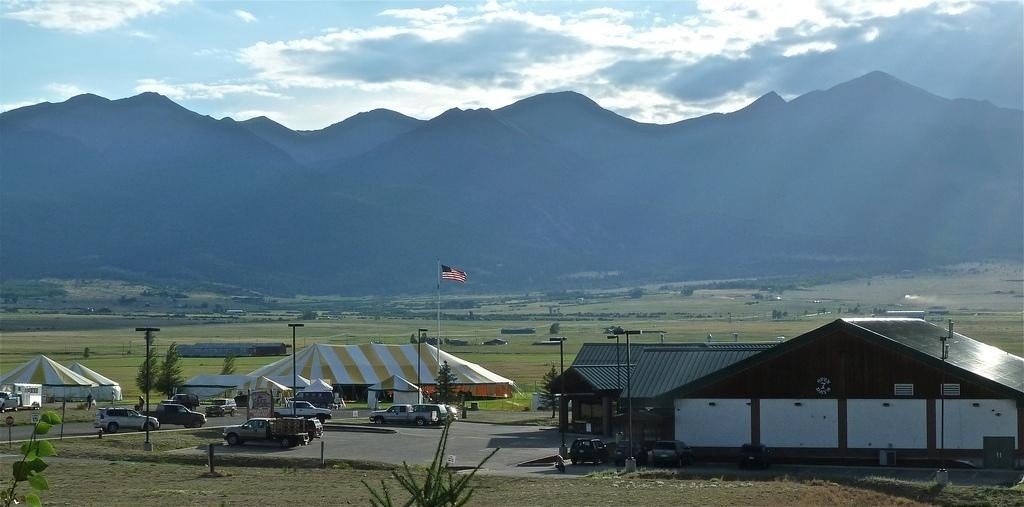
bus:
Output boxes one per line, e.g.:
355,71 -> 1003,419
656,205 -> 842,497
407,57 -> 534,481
1,383 -> 42,413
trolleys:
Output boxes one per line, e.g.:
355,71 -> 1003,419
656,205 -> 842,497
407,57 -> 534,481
135,405 -> 142,411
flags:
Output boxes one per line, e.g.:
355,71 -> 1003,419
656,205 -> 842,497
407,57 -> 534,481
439,263 -> 465,282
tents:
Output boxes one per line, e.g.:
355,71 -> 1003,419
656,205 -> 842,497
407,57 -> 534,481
0,353 -> 99,403
65,361 -> 122,401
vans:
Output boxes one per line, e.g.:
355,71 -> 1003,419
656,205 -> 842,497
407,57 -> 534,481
289,391 -> 333,408
570,438 -> 610,464
412,404 -> 458,425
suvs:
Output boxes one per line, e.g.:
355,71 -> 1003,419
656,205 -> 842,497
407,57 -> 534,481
94,407 -> 159,433
206,397 -> 238,417
161,393 -> 200,409
306,418 -> 323,441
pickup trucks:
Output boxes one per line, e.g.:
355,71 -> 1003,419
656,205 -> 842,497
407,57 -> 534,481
223,417 -> 310,448
142,403 -> 207,428
268,401 -> 332,423
369,404 -> 438,426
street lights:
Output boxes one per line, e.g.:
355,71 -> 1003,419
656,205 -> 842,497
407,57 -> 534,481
938,318 -> 954,471
550,337 -> 567,446
418,328 -> 428,410
135,327 -> 160,443
607,329 -> 642,459
288,323 -> 304,417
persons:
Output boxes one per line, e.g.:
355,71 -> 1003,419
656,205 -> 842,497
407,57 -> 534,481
86,391 -> 93,411
138,395 -> 143,409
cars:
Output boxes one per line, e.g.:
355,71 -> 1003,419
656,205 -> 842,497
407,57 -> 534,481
482,339 -> 508,345
0,383 -> 20,413
614,440 -> 696,468
447,339 -> 467,346
234,391 -> 275,408
738,443 -> 773,468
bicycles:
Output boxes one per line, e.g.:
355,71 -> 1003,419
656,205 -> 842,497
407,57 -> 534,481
553,455 -> 565,473
